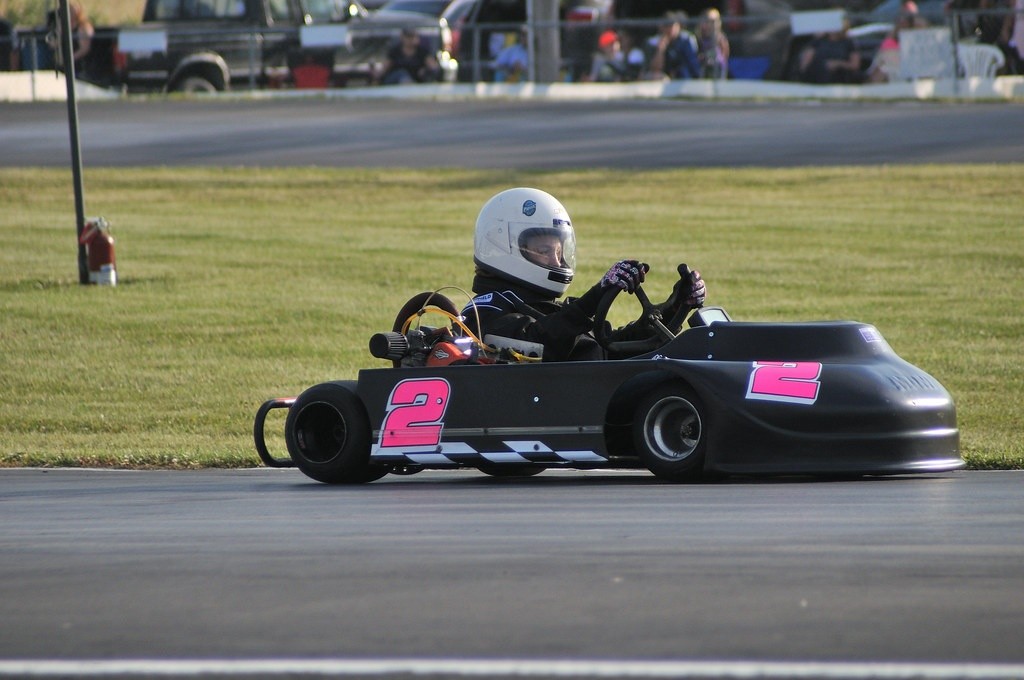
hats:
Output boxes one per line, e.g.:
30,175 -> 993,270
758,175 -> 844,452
598,30 -> 620,48
660,11 -> 679,27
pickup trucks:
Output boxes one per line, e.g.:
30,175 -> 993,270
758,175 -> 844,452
14,0 -> 368,95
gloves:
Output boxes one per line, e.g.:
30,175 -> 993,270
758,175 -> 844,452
674,270 -> 707,310
600,260 -> 650,294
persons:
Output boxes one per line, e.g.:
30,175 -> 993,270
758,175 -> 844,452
465,187 -> 707,358
0,0 -> 25,72
582,8 -> 730,82
800,0 -> 1024,86
380,27 -> 437,84
496,23 -> 531,80
45,0 -> 93,80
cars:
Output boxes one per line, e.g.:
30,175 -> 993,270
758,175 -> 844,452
438,0 -> 573,82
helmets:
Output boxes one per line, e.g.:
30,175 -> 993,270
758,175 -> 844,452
473,187 -> 578,301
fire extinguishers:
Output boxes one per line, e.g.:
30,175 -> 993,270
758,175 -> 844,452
79,217 -> 118,286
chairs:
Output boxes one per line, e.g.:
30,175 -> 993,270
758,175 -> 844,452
269,30 -> 1024,85
